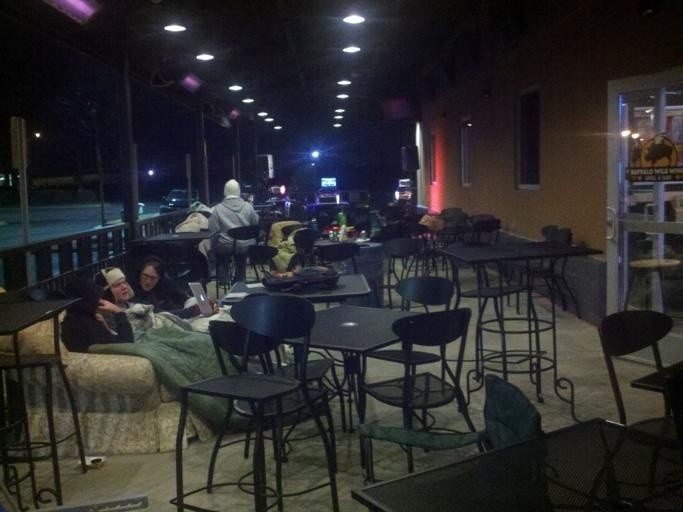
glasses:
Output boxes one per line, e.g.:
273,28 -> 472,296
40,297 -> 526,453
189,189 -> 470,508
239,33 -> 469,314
142,273 -> 161,282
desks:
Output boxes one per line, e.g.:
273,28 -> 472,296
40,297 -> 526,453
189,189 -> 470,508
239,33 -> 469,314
0,295 -> 87,503
128,229 -> 215,284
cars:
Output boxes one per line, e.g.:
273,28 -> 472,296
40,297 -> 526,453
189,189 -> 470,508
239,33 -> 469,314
160,189 -> 196,212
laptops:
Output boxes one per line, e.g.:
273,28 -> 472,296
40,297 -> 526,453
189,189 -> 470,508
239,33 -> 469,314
184,281 -> 214,323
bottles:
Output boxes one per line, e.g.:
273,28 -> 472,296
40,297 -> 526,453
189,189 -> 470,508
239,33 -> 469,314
328,224 -> 367,243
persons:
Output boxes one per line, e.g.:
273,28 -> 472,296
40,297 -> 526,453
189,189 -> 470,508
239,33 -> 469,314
60,278 -> 133,351
366,202 -> 407,243
125,255 -> 219,320
93,267 -> 168,333
205,177 -> 259,291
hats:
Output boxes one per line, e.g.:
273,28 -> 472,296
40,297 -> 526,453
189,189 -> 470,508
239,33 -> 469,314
94,266 -> 135,305
224,179 -> 241,199
66,276 -> 106,302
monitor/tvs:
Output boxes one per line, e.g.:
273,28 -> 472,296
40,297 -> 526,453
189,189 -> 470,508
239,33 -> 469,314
320,177 -> 337,188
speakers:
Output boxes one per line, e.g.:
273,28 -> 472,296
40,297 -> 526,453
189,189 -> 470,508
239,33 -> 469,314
399,143 -> 418,171
256,153 -> 275,180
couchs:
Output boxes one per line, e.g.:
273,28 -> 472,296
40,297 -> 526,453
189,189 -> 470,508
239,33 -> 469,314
0,289 -> 210,453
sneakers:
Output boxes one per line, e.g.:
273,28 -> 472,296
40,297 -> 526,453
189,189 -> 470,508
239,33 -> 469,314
217,282 -> 228,289
